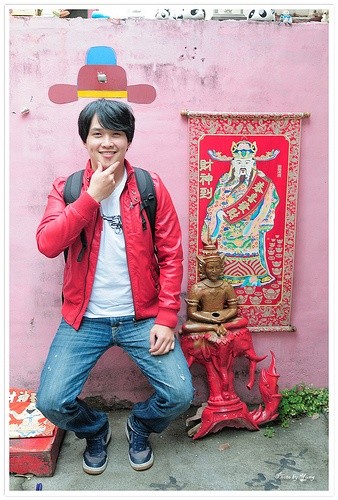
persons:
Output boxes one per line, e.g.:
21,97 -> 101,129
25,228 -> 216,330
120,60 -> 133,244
183,253 -> 249,336
35,98 -> 193,476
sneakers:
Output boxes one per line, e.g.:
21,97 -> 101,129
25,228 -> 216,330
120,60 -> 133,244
125,418 -> 154,471
83,427 -> 112,474
